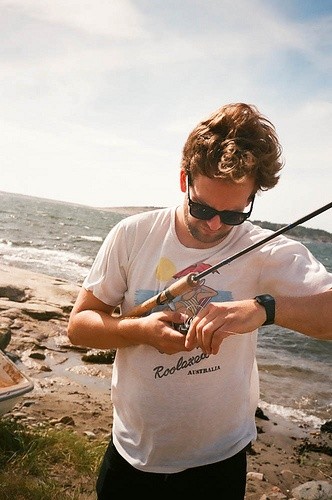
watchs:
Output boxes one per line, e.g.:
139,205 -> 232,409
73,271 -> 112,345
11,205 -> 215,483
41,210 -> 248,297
253,293 -> 275,325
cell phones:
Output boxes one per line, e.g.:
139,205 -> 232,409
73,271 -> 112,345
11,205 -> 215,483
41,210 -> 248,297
174,323 -> 190,333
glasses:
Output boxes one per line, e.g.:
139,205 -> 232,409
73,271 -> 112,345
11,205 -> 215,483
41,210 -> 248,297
187,170 -> 255,225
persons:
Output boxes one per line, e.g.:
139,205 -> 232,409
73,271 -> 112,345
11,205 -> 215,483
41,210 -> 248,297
68,102 -> 332,500
